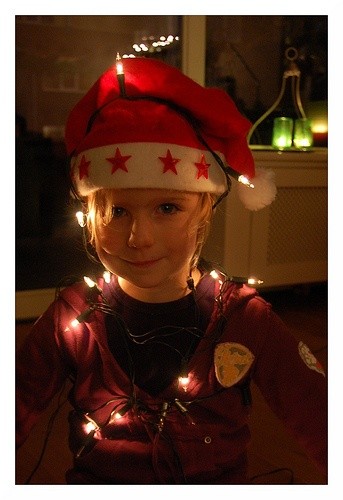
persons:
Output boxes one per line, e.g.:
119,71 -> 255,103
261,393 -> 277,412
15,58 -> 328,484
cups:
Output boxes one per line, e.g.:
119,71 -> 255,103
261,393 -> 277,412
294,117 -> 312,148
272,116 -> 293,150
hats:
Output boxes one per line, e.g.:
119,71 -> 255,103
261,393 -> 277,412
63,56 -> 275,211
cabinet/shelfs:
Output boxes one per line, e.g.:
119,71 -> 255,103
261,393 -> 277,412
200,148 -> 327,288
